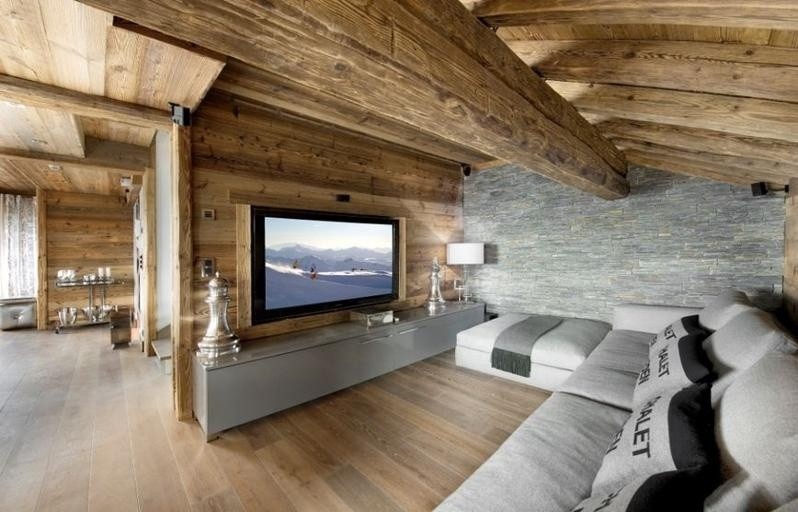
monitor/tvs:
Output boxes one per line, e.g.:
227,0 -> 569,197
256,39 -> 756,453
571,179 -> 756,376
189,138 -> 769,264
250,204 -> 399,326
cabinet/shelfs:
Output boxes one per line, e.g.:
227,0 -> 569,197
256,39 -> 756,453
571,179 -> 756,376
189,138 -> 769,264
192,301 -> 486,444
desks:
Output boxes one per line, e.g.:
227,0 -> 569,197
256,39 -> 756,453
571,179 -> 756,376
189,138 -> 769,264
55,279 -> 118,334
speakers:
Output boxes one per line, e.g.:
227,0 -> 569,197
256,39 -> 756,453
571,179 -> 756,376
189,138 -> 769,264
173,106 -> 191,127
751,181 -> 767,196
462,165 -> 471,177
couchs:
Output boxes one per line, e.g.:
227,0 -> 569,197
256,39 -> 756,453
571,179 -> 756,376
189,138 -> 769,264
453,302 -> 797,512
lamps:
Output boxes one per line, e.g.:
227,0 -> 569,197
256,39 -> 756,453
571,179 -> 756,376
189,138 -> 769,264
446,243 -> 485,303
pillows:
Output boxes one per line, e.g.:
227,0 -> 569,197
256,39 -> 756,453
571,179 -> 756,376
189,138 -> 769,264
571,315 -> 721,511
